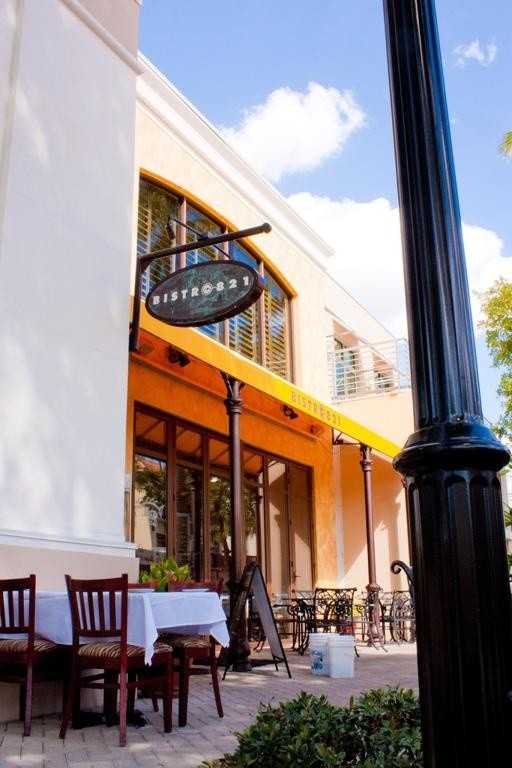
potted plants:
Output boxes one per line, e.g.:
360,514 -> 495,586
139,558 -> 194,696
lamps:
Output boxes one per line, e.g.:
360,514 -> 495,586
312,425 -> 324,436
137,338 -> 154,354
283,405 -> 299,420
169,349 -> 191,367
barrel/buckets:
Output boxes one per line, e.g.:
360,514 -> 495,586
329,636 -> 355,678
308,633 -> 340,675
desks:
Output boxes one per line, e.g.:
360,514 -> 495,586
0,591 -> 229,728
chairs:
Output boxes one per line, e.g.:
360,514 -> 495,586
0,574 -> 73,736
59,574 -> 174,747
128,580 -> 159,712
248,587 -> 418,659
127,578 -> 225,727
